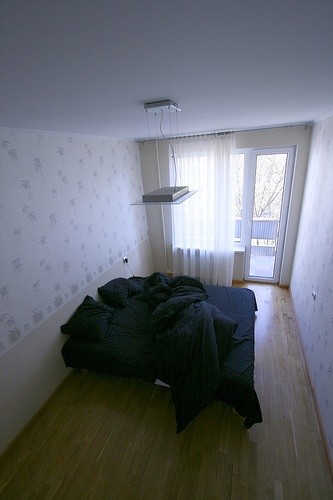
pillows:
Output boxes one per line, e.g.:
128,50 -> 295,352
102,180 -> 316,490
61,295 -> 114,341
98,278 -> 141,309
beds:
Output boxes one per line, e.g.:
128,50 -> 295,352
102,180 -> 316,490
61,278 -> 263,429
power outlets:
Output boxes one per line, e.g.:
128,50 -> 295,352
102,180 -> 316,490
123,256 -> 128,263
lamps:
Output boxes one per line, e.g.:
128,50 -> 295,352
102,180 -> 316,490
130,101 -> 198,206
